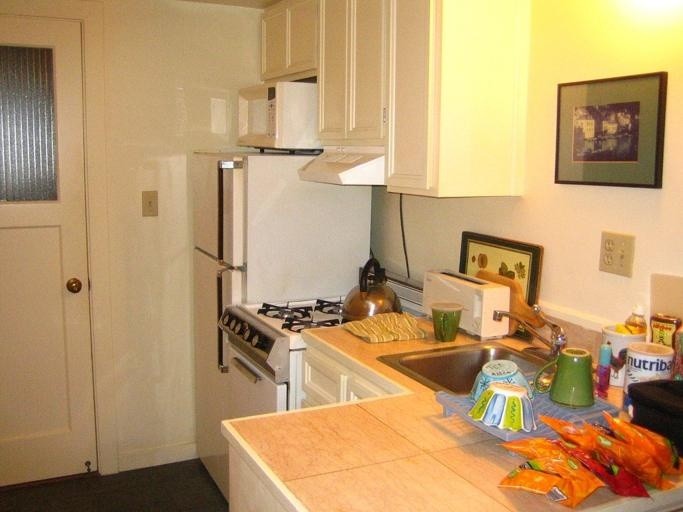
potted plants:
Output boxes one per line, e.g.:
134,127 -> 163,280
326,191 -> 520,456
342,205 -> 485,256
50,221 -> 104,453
552,71 -> 667,188
458,229 -> 545,308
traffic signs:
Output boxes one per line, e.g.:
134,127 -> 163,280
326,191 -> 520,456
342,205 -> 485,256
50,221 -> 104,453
596,231 -> 637,279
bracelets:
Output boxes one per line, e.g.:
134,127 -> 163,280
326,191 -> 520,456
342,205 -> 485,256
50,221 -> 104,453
467,359 -> 535,401
467,382 -> 537,433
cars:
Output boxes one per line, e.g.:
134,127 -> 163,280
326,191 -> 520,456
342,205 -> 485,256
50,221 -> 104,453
602,325 -> 647,387
534,348 -> 595,407
431,302 -> 464,342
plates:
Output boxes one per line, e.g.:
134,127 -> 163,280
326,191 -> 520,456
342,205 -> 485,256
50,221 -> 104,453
255,1 -> 318,84
387,0 -> 529,199
299,349 -> 387,409
316,0 -> 388,146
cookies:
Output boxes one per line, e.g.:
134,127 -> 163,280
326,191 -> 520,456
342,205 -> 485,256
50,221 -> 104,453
235,81 -> 323,153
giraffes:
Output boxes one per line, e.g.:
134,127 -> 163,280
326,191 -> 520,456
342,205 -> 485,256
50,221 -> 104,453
257,296 -> 352,333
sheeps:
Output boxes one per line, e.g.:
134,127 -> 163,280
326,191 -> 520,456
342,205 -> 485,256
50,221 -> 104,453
492,303 -> 567,359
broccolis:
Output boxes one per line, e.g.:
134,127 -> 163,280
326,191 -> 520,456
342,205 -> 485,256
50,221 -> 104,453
192,151 -> 372,504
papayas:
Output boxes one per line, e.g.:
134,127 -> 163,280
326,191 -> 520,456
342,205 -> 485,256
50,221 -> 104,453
421,267 -> 510,341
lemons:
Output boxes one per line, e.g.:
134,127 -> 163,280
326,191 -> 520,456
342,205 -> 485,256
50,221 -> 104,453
341,258 -> 403,321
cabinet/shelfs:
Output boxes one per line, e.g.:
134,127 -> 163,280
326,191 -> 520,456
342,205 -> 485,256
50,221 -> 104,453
596,344 -> 612,391
625,304 -> 647,330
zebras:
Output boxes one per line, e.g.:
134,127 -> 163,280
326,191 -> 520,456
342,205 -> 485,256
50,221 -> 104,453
226,343 -> 287,420
